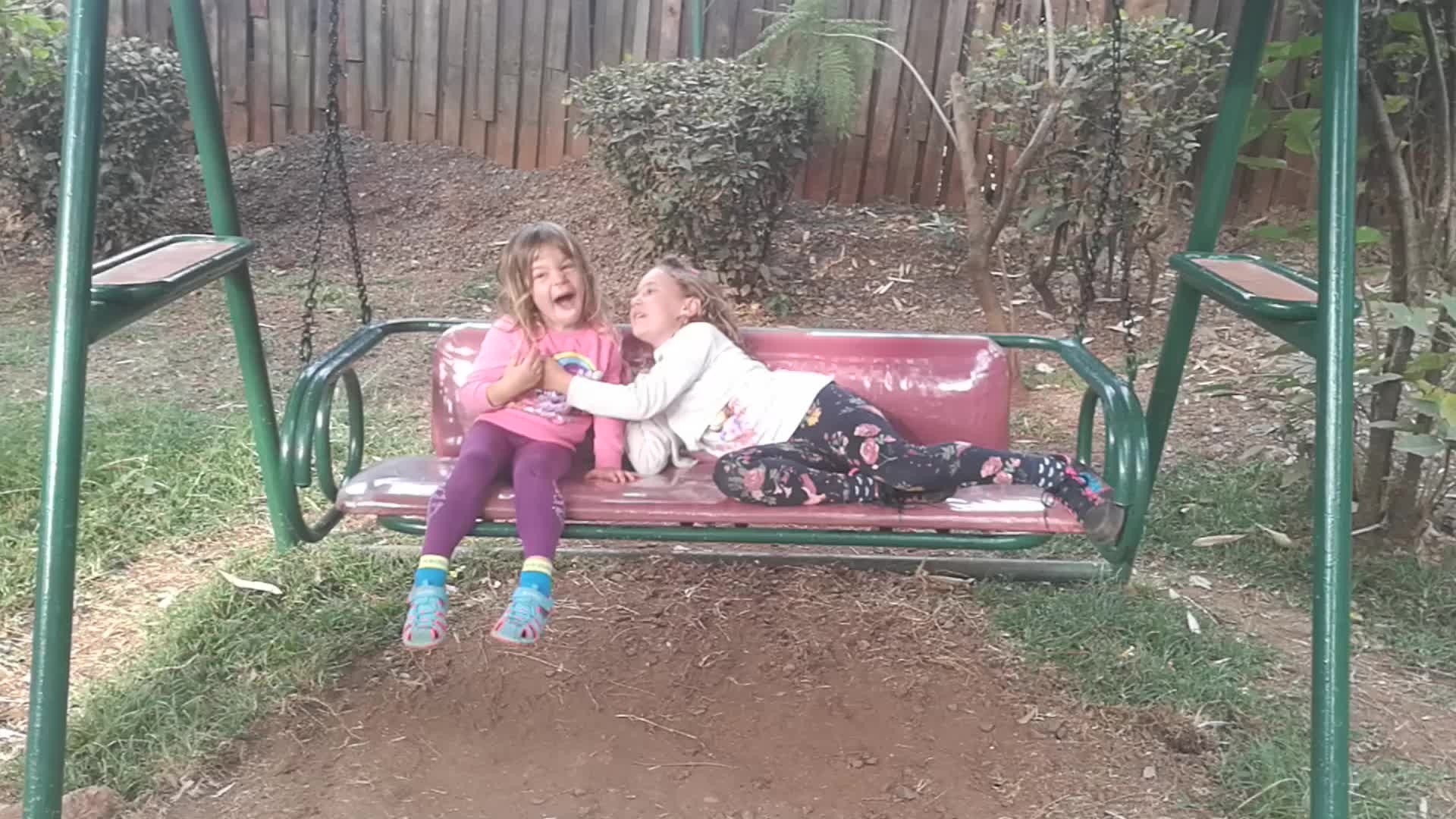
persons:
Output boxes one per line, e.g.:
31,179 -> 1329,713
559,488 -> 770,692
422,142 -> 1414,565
537,261 -> 1123,542
402,220 -> 643,650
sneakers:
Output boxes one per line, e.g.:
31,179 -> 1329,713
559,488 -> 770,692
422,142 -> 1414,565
1042,453 -> 1127,543
894,487 -> 955,504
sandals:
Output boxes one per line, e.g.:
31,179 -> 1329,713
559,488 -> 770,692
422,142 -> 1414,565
489,588 -> 554,643
402,586 -> 451,650
